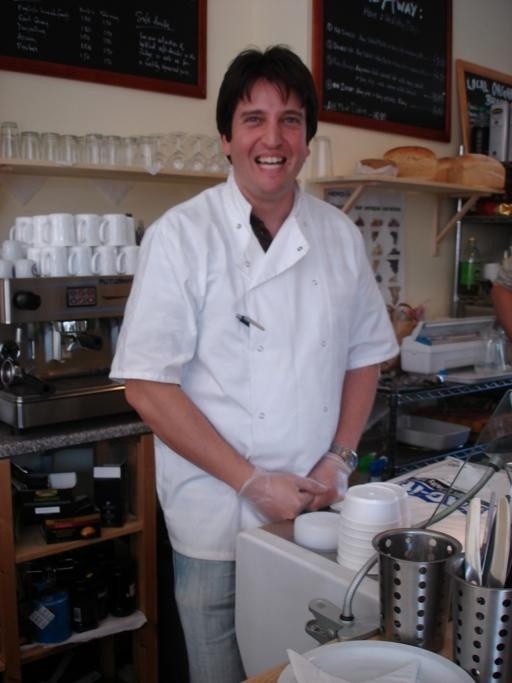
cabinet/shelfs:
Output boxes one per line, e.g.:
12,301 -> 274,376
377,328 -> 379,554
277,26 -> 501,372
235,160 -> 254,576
1,435 -> 161,682
373,377 -> 512,477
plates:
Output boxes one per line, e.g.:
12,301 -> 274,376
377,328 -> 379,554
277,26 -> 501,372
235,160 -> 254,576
276,639 -> 476,683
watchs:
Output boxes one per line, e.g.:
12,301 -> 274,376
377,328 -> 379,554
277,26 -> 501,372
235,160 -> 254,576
329,444 -> 360,471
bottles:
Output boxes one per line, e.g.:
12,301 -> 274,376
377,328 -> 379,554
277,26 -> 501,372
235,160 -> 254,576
469,125 -> 488,152
460,237 -> 480,293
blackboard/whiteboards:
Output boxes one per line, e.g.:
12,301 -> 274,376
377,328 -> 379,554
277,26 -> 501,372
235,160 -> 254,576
0,0 -> 207,99
311,0 -> 453,143
456,59 -> 512,214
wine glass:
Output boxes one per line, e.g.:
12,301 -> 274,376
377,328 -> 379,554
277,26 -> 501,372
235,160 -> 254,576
158,131 -> 227,172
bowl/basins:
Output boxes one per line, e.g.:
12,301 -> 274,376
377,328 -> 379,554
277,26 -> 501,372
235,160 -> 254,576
335,480 -> 409,577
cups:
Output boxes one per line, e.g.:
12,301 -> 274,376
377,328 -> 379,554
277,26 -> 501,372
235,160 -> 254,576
484,261 -> 500,281
474,325 -> 505,375
1,212 -> 142,276
310,139 -> 333,178
0,121 -> 156,168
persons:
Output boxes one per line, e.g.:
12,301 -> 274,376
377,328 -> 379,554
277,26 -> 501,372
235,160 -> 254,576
108,46 -> 400,683
490,245 -> 512,341
